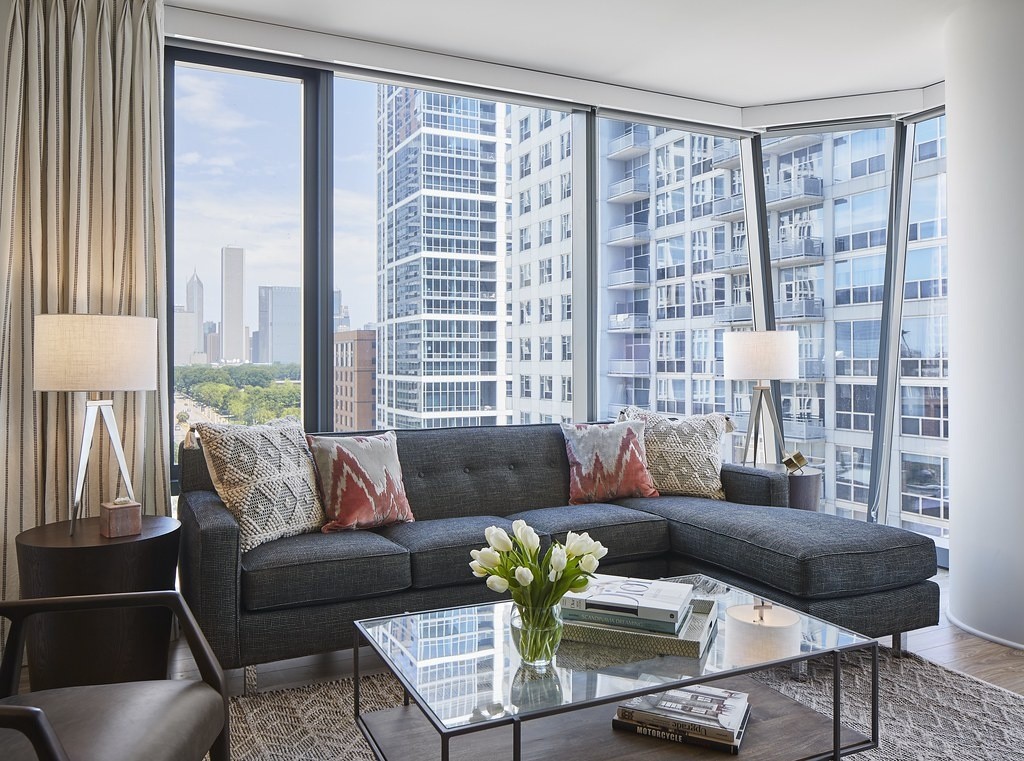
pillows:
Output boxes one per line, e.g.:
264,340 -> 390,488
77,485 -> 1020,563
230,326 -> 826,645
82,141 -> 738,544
182,414 -> 329,554
615,405 -> 738,502
307,430 -> 415,534
560,421 -> 660,504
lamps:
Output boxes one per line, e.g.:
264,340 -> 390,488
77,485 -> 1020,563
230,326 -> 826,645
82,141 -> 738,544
33,313 -> 161,535
722,332 -> 800,466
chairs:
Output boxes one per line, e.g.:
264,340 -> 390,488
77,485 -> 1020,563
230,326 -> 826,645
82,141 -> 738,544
0,591 -> 234,761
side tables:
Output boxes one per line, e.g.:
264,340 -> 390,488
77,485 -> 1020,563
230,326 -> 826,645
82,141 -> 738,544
749,462 -> 821,514
16,514 -> 182,693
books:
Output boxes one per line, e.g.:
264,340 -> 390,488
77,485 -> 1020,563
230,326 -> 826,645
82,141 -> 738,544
597,620 -> 717,684
559,573 -> 717,658
612,673 -> 753,755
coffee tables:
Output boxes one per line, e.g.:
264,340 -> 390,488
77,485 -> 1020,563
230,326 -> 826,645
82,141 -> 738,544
353,573 -> 881,761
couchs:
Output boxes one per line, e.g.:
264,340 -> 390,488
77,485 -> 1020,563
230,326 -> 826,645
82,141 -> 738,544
177,424 -> 941,674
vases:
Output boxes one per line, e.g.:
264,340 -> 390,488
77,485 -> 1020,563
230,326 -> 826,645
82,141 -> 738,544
509,602 -> 565,664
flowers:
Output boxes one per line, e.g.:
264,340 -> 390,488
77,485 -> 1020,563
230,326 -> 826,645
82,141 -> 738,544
469,518 -> 609,660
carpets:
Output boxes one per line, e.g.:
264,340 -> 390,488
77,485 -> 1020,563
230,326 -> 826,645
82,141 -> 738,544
202,645 -> 1024,761
